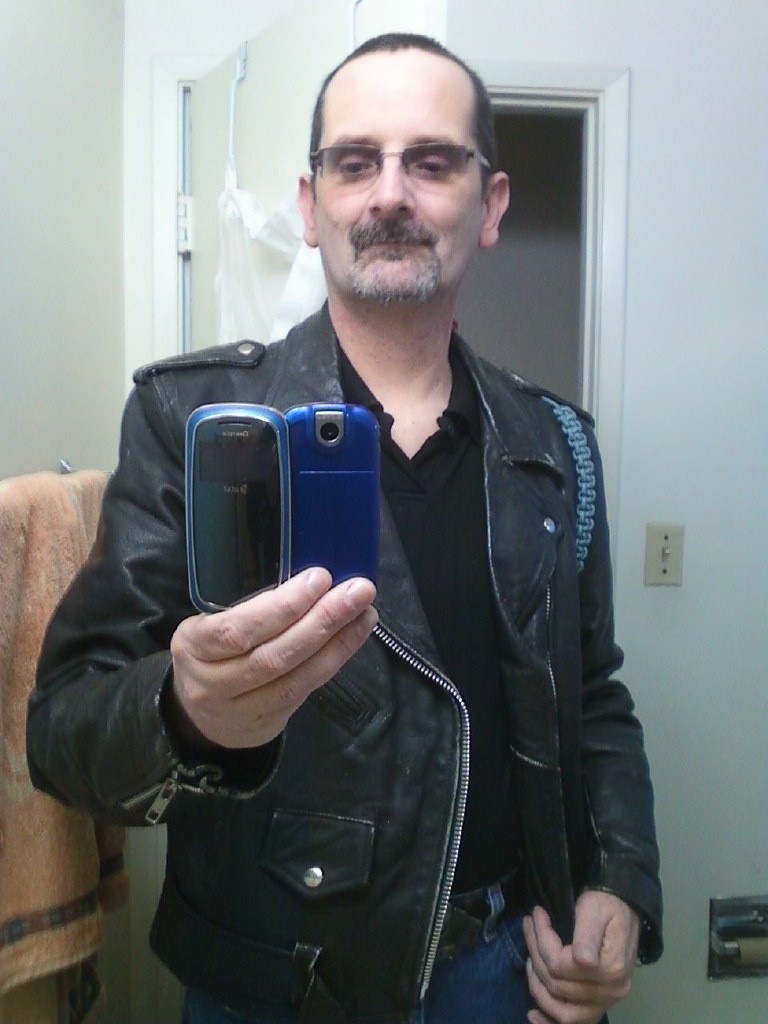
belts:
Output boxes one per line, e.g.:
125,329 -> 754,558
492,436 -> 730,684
437,873 -> 529,957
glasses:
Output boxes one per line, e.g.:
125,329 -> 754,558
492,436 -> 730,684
309,138 -> 492,182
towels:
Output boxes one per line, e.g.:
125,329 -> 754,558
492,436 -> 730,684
1,467 -> 131,1023
218,186 -> 328,347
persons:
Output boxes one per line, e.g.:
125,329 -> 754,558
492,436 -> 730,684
27,33 -> 665,1023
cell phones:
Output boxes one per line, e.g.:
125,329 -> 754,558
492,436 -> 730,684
185,401 -> 381,613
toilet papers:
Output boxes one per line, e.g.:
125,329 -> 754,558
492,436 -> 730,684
734,935 -> 768,967
